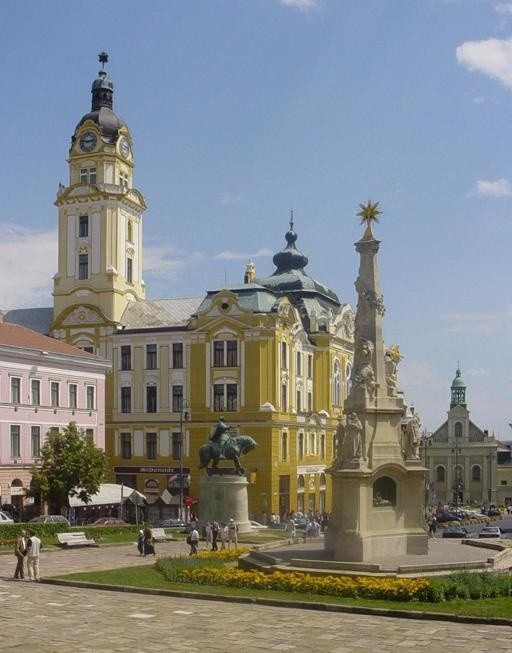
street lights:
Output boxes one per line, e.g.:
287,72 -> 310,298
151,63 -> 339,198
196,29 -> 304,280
451,436 -> 461,505
485,446 -> 497,503
419,429 -> 433,520
179,399 -> 191,523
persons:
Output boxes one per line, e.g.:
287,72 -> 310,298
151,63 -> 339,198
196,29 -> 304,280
137,529 -> 143,555
13,527 -> 27,579
258,509 -> 332,545
184,517 -> 242,557
211,416 -> 231,459
404,418 -> 420,460
142,524 -> 156,556
425,501 -> 512,536
23,529 -> 42,581
341,413 -> 362,460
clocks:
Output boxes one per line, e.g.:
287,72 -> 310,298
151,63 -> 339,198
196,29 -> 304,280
79,130 -> 98,152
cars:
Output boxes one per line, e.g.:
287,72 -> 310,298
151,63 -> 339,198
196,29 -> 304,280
442,527 -> 469,538
435,505 -> 485,522
0,511 -> 15,523
274,517 -> 309,529
248,520 -> 269,529
28,515 -> 70,527
90,517 -> 125,526
479,526 -> 502,537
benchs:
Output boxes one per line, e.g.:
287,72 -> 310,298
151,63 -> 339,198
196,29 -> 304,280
55,531 -> 96,549
139,527 -> 173,542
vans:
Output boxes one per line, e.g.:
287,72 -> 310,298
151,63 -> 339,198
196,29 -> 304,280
160,519 -> 186,526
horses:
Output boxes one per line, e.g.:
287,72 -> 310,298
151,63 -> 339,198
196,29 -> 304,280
197,434 -> 259,477
